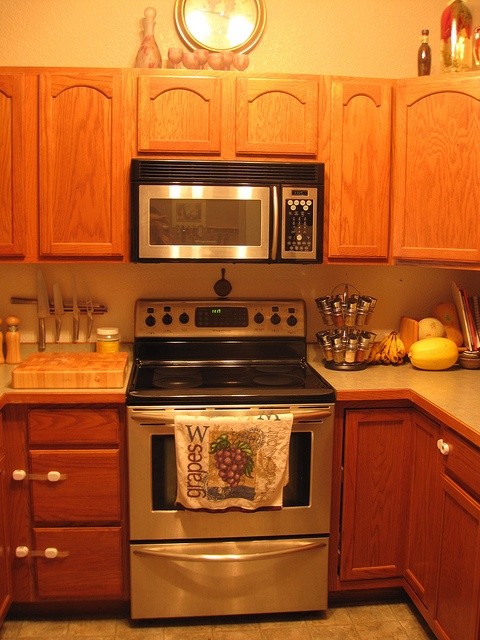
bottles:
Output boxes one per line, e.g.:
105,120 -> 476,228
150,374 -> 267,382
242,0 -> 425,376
96,327 -> 120,352
5,314 -> 24,365
315,329 -> 377,363
0,316 -> 5,367
315,294 -> 377,326
416,29 -> 431,75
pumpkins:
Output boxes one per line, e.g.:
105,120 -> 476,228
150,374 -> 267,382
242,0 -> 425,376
432,301 -> 464,347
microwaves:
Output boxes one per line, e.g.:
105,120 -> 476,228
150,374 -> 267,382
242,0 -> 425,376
136,177 -> 320,265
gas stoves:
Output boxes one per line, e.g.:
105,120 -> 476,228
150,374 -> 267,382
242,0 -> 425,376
129,298 -> 336,405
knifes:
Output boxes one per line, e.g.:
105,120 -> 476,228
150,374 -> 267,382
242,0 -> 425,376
70,291 -> 80,345
85,286 -> 95,339
34,269 -> 50,353
50,282 -> 67,346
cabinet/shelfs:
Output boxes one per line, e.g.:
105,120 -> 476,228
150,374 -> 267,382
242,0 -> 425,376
131,69 -> 327,158
340,402 -> 443,609
437,423 -> 478,640
326,74 -> 392,265
388,73 -> 480,269
0,64 -> 133,271
0,403 -> 118,621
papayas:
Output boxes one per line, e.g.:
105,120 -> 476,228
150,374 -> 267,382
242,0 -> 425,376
408,337 -> 458,371
417,318 -> 445,339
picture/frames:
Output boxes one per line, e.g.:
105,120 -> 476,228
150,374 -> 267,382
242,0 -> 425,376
175,1 -> 266,58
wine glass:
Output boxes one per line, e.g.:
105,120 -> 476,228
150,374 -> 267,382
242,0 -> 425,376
209,51 -> 224,70
183,53 -> 198,69
235,54 -> 248,72
168,47 -> 182,68
198,48 -> 209,71
224,51 -> 234,73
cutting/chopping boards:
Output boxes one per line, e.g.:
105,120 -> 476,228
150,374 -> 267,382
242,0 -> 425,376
10,351 -> 131,388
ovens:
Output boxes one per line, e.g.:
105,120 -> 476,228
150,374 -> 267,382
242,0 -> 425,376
128,406 -> 333,621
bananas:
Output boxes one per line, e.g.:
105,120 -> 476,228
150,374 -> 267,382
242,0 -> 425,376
366,331 -> 406,364
